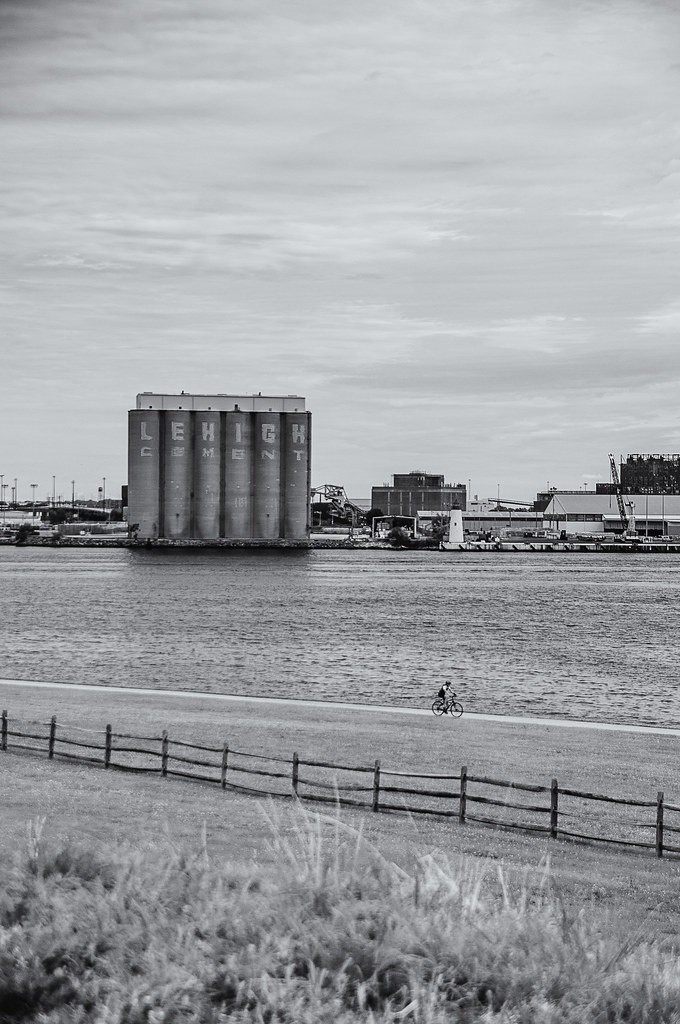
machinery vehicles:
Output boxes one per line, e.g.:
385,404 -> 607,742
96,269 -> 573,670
608,453 -> 639,541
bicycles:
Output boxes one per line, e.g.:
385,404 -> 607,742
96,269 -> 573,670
431,695 -> 464,719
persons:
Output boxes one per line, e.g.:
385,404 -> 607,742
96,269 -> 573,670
438,680 -> 457,714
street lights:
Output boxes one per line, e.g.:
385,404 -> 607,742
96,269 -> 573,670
498,483 -> 500,499
468,479 -> 471,500
103,477 -> 106,509
53,475 -> 56,501
29,484 -> 38,500
0,473 -> 15,501
71,479 -> 75,510
14,478 -> 17,502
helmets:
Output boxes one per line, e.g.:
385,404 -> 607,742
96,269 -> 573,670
446,680 -> 451,684
